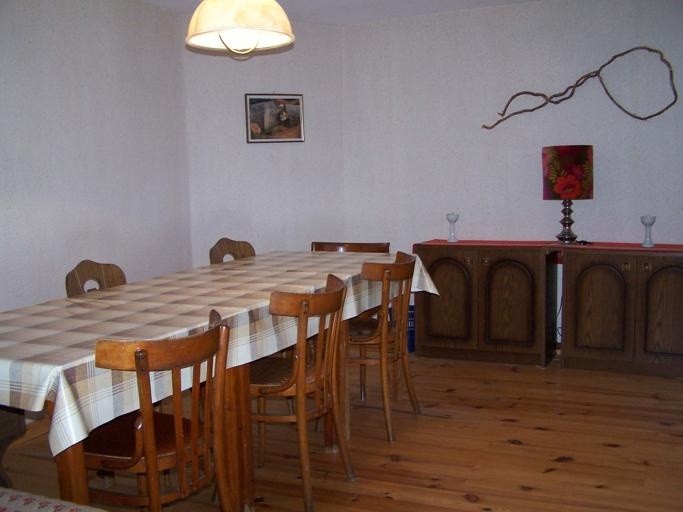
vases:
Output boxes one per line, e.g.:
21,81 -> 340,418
446,212 -> 461,243
638,215 -> 659,248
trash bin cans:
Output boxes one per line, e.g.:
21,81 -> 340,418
388,305 -> 415,352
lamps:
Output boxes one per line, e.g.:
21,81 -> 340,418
540,143 -> 594,244
181,0 -> 297,59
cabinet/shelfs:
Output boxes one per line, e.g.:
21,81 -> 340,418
411,238 -> 561,368
557,241 -> 682,380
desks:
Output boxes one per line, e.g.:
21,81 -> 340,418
0,248 -> 441,512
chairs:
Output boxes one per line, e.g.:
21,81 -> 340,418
207,236 -> 297,425
188,272 -> 356,512
309,241 -> 392,401
53,302 -> 238,511
315,250 -> 422,450
62,255 -> 128,297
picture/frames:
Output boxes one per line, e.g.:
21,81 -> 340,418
242,92 -> 308,145
